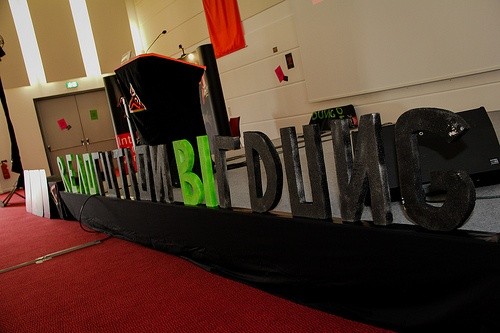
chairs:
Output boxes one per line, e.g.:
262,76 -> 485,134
229,117 -> 240,137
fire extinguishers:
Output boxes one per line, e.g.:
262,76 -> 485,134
1,160 -> 11,180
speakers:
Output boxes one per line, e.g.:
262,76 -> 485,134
351,106 -> 500,207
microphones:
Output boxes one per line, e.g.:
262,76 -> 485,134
146,30 -> 166,53
